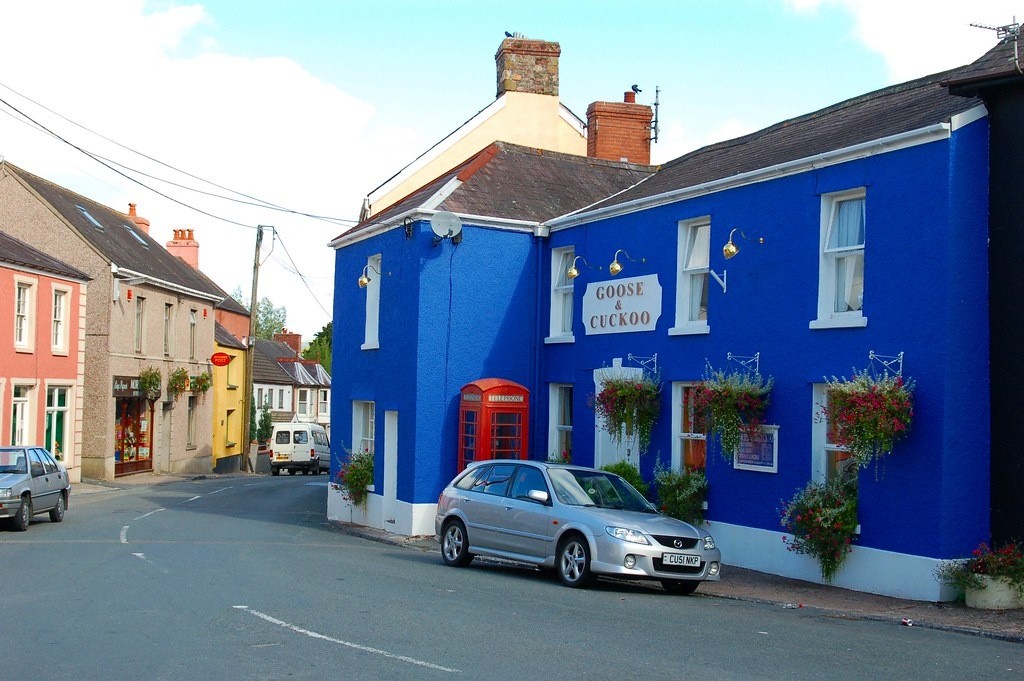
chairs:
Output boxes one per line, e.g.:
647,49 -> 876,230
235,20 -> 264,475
17,457 -> 27,467
512,471 -> 534,497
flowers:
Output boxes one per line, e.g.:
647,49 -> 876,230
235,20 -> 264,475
776,465 -> 859,584
931,542 -> 1024,601
814,366 -> 916,480
587,361 -> 663,456
681,365 -> 774,459
653,450 -> 708,525
550,449 -> 571,463
331,440 -> 374,510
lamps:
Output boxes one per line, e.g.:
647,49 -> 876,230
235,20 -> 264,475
610,250 -> 645,276
359,265 -> 391,288
723,228 -> 763,258
567,256 -> 602,280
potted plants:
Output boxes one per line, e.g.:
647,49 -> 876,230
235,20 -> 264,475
196,372 -> 212,390
169,367 -> 189,393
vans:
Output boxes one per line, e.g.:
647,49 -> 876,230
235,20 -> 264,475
269,422 -> 329,476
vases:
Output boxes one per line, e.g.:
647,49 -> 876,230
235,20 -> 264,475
965,573 -> 1024,610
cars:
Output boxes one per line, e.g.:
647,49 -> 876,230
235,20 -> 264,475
0,445 -> 73,531
433,461 -> 722,596
